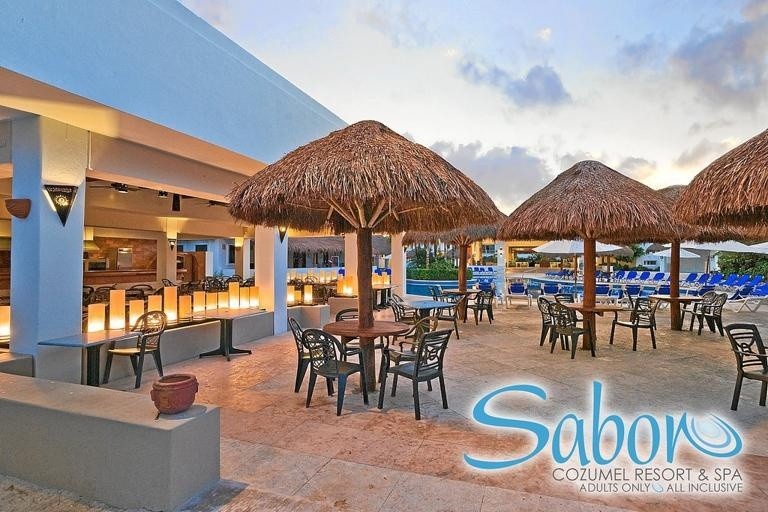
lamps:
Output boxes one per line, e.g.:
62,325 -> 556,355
118,187 -> 128,194
0,305 -> 11,337
158,191 -> 169,198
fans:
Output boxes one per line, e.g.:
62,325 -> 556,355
200,201 -> 231,208
89,183 -> 142,194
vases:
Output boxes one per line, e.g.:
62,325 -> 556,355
149,374 -> 199,414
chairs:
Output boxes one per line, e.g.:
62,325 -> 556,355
37,330 -> 141,387
83,274 -> 255,389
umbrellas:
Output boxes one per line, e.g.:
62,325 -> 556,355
681,239 -> 756,289
532,238 -> 623,304
230,115 -> 511,392
653,247 -> 701,260
748,240 -> 768,256
615,179 -> 768,332
677,120 -> 767,232
496,159 -> 677,350
401,210 -> 510,320
